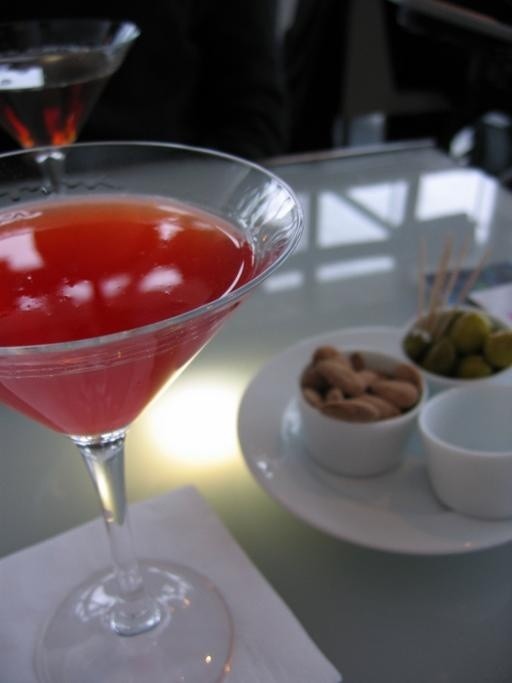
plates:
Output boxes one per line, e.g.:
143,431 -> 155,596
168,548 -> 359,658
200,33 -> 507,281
238,325 -> 511,556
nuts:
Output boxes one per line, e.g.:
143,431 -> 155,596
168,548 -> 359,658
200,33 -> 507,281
301,342 -> 421,421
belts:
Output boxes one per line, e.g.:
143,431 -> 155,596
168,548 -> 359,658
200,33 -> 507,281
0,14 -> 144,194
0,139 -> 307,683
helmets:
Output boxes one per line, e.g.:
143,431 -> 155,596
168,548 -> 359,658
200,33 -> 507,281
238,325 -> 511,556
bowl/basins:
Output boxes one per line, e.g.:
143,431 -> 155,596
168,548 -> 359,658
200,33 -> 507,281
399,312 -> 511,393
418,380 -> 511,520
294,346 -> 426,482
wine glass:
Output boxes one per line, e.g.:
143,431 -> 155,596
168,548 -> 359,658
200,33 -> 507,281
0,14 -> 144,194
0,139 -> 307,683
